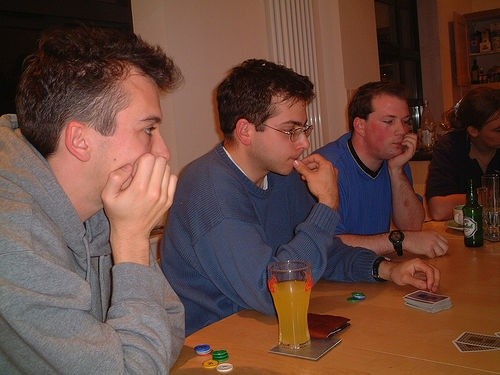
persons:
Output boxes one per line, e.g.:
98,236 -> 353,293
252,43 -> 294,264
162,59 -> 440,342
0,23 -> 185,374
425,85 -> 500,221
310,81 -> 448,259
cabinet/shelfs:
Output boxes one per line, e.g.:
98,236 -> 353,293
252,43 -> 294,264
452,7 -> 500,91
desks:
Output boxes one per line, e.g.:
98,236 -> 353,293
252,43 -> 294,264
169,220 -> 499,375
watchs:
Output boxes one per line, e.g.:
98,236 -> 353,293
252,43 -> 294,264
388,230 -> 404,256
372,255 -> 391,282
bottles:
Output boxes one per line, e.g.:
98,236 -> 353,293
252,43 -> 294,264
463,179 -> 483,247
421,101 -> 435,151
471,60 -> 479,84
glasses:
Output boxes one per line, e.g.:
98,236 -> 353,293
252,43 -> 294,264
243,115 -> 314,142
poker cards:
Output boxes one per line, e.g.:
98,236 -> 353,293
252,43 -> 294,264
402,288 -> 453,314
451,329 -> 500,354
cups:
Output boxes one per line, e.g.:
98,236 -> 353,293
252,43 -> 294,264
481,174 -> 500,242
267,260 -> 314,350
453,205 -> 464,225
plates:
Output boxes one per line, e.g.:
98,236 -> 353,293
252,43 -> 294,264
447,222 -> 463,231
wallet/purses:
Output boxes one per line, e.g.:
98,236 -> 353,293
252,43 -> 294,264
307,312 -> 350,339
